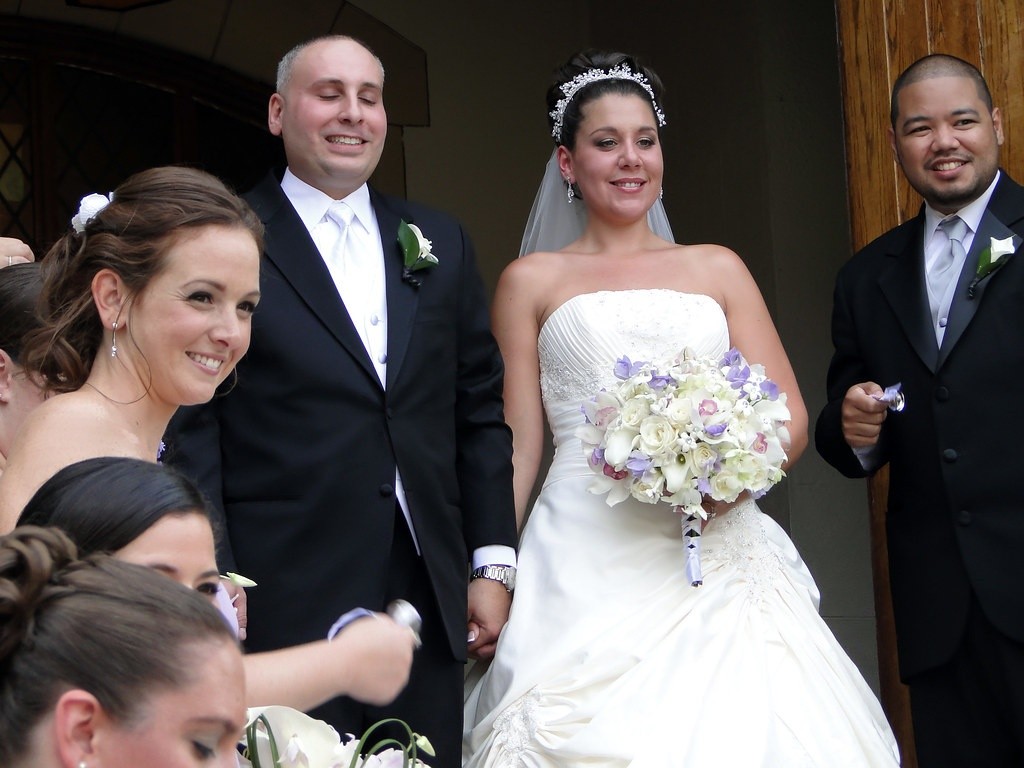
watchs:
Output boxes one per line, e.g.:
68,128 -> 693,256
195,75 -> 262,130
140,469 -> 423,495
470,565 -> 518,594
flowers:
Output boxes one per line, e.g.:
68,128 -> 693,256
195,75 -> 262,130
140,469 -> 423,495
398,219 -> 440,289
243,705 -> 435,768
575,348 -> 792,588
69,191 -> 114,234
969,234 -> 1017,290
219,572 -> 257,587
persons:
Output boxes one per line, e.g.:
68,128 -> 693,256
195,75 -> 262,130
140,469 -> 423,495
158,35 -> 519,766
463,56 -> 901,768
0,166 -> 420,768
814,54 -> 1024,768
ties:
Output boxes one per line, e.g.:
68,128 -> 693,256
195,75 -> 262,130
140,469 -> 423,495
927,218 -> 968,303
325,201 -> 378,320
